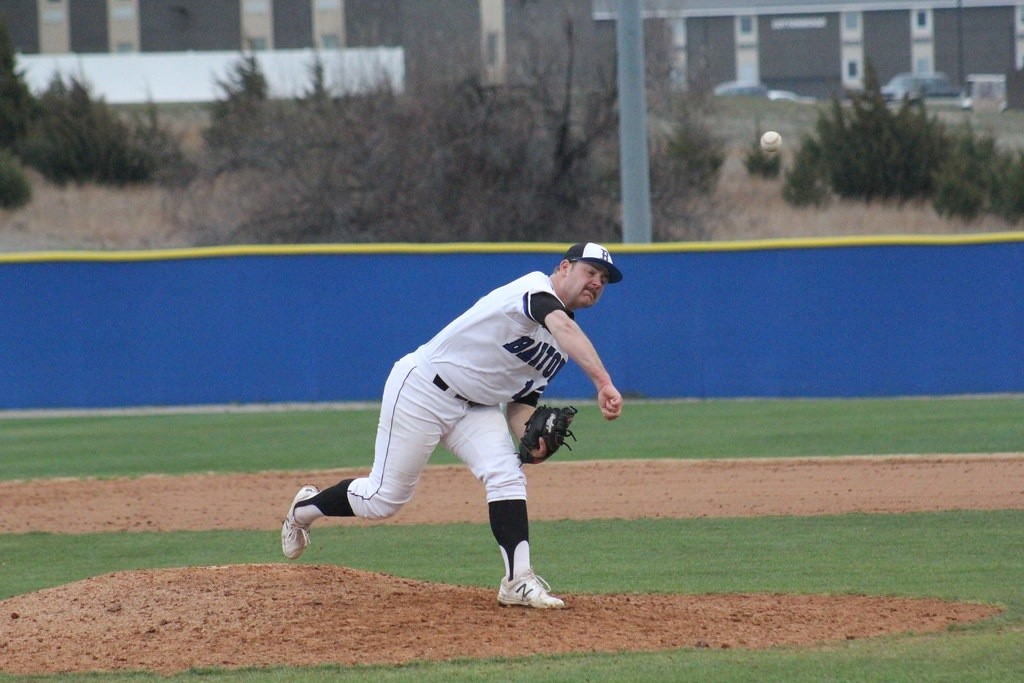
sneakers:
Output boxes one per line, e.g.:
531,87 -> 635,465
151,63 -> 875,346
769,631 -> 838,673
281,484 -> 320,558
497,566 -> 565,608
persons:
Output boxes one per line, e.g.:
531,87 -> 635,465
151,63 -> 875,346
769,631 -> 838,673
281,242 -> 623,610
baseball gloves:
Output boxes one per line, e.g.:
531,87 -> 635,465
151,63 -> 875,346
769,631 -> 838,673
512,403 -> 579,469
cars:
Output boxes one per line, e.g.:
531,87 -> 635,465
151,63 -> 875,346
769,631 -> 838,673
714,82 -> 798,102
881,72 -> 958,103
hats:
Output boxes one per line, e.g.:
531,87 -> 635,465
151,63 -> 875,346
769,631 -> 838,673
559,242 -> 623,284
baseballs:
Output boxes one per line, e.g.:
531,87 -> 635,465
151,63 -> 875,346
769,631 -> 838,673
761,131 -> 783,153
